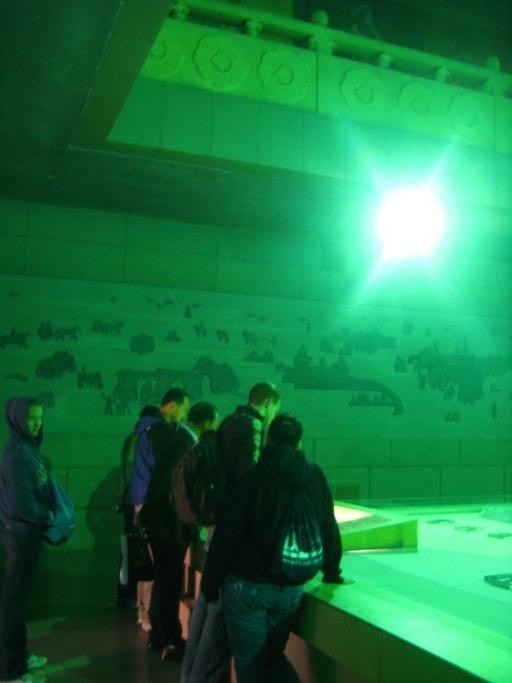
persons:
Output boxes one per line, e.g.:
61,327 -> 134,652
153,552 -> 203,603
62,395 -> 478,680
0,392 -> 56,683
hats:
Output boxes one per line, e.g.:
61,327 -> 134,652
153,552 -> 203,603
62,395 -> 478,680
1,654 -> 49,683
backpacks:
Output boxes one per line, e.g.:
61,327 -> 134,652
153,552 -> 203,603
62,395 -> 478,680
252,488 -> 328,590
18,440 -> 77,546
183,428 -> 228,529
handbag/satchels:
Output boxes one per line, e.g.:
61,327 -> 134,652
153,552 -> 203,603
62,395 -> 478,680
118,532 -> 149,581
